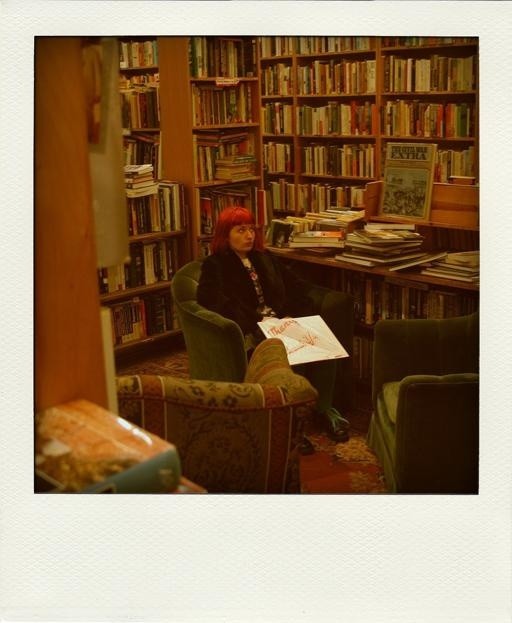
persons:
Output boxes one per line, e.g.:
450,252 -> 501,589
194,204 -> 350,455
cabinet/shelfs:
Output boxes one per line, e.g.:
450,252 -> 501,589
97,35 -> 479,389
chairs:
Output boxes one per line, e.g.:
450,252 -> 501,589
114,339 -> 319,493
366,312 -> 478,494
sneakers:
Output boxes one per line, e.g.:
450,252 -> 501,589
299,437 -> 315,456
314,408 -> 352,443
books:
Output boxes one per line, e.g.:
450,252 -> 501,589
34,398 -> 181,493
92,38 -> 186,346
187,35 -> 478,282
256,314 -> 348,366
328,268 -> 479,379
